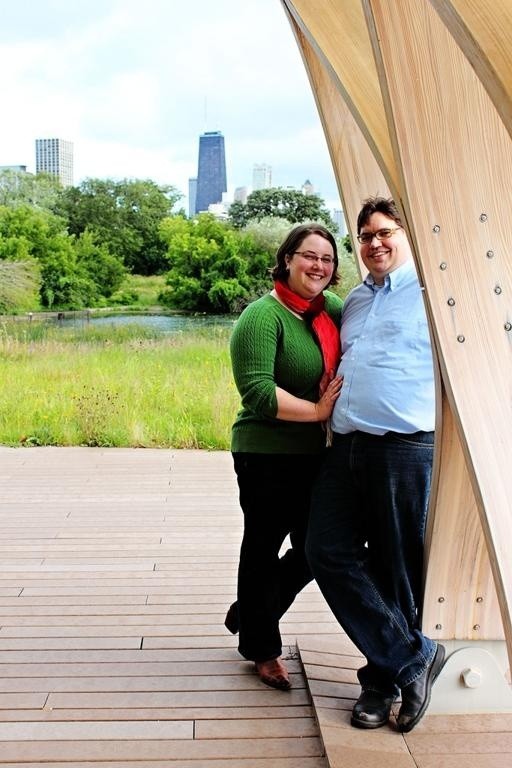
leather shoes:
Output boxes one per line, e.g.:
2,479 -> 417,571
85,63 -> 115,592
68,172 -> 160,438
255,656 -> 292,689
397,643 -> 445,733
351,689 -> 393,729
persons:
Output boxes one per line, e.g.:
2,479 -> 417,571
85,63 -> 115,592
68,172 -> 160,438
220,224 -> 350,694
301,193 -> 452,735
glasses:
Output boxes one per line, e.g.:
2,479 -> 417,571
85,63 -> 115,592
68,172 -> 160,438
294,250 -> 334,264
357,227 -> 402,244
